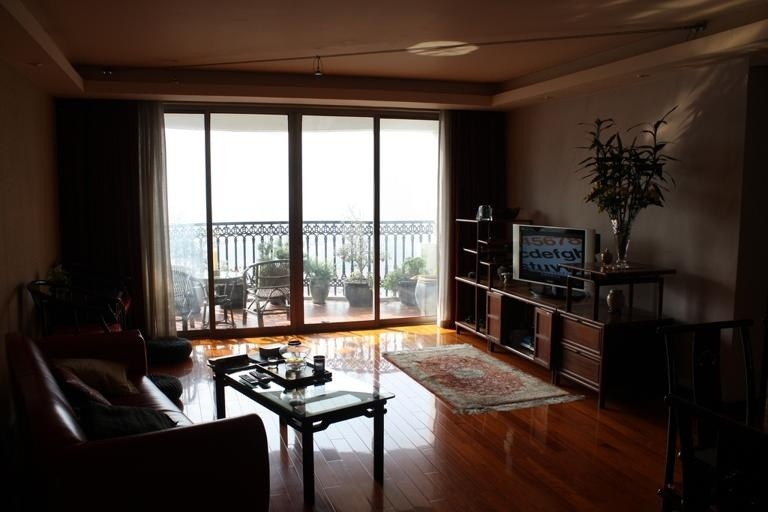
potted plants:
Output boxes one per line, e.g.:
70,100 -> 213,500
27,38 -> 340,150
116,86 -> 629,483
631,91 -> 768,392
575,100 -> 682,270
47,263 -> 69,295
310,235 -> 426,308
258,243 -> 290,306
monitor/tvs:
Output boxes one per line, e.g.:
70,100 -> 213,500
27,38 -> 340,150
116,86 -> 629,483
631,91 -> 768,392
512,224 -> 596,300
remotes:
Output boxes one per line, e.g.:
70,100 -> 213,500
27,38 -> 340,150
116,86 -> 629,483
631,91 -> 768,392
250,371 -> 271,383
239,374 -> 257,385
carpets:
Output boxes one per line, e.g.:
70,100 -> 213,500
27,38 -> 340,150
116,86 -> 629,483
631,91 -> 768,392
382,342 -> 585,417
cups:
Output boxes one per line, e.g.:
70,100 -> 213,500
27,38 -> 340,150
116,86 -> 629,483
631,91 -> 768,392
313,355 -> 325,376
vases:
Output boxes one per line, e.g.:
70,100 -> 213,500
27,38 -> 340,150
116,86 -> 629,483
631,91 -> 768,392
415,274 -> 438,316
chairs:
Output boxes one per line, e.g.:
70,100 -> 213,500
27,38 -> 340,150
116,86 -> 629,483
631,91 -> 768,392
170,260 -> 294,332
27,281 -> 127,332
656,318 -> 767,510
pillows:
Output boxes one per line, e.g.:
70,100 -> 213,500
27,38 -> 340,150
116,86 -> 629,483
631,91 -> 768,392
49,353 -> 135,406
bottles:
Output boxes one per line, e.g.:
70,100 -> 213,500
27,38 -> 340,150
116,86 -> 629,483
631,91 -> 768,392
475,202 -> 493,222
278,340 -> 312,372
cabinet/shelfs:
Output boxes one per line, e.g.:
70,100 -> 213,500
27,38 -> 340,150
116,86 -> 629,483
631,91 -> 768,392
454,217 -> 678,412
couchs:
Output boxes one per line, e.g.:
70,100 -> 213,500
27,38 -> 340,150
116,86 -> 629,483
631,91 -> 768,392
6,328 -> 271,509
